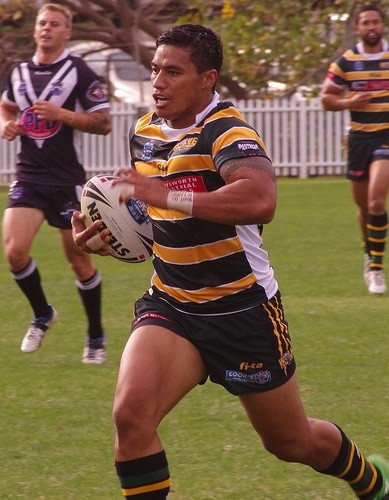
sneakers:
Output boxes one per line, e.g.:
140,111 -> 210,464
82,336 -> 106,364
368,270 -> 386,293
20,305 -> 59,354
364,257 -> 370,285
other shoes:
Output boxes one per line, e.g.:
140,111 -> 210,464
364,452 -> 389,500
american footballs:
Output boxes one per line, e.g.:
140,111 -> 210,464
80,172 -> 155,263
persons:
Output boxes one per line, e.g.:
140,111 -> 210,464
71,25 -> 389,499
0,3 -> 113,365
319,4 -> 389,295
